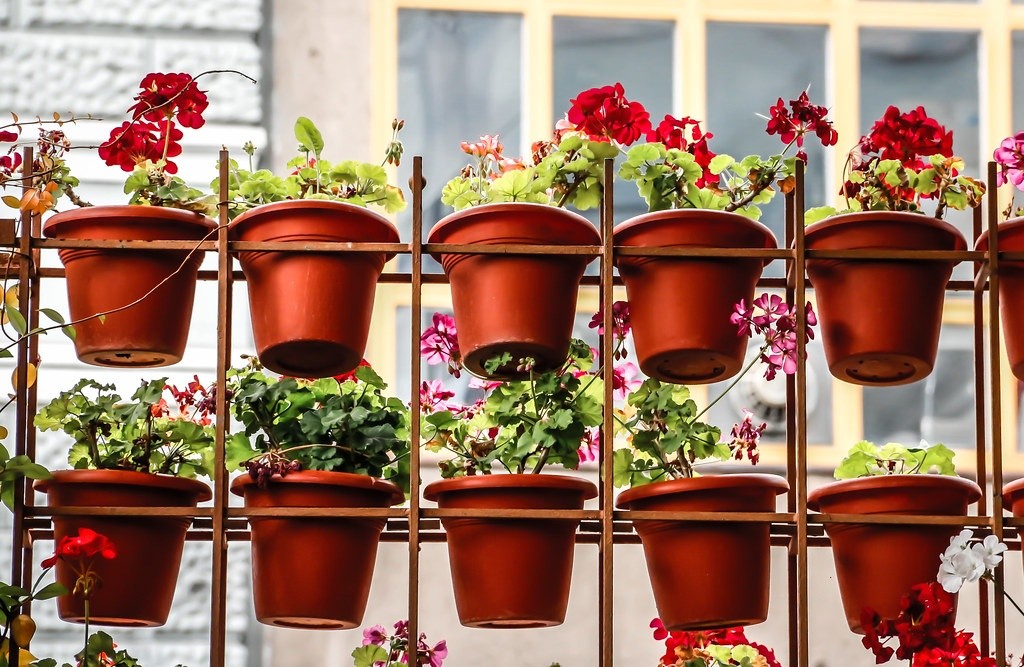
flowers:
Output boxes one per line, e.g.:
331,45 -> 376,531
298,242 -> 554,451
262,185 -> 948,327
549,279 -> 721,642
0,68 -> 1024,667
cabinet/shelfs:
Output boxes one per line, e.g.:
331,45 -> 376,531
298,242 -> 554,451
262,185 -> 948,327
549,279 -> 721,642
0,146 -> 1024,666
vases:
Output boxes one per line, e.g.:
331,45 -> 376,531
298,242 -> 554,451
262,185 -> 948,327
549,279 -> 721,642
807,474 -> 983,636
1000,479 -> 1024,549
615,474 -> 791,632
613,209 -> 778,384
974,217 -> 1024,383
424,474 -> 597,630
228,199 -> 400,378
427,203 -> 601,381
31,468 -> 212,628
228,472 -> 401,630
789,211 -> 969,388
43,206 -> 219,368
